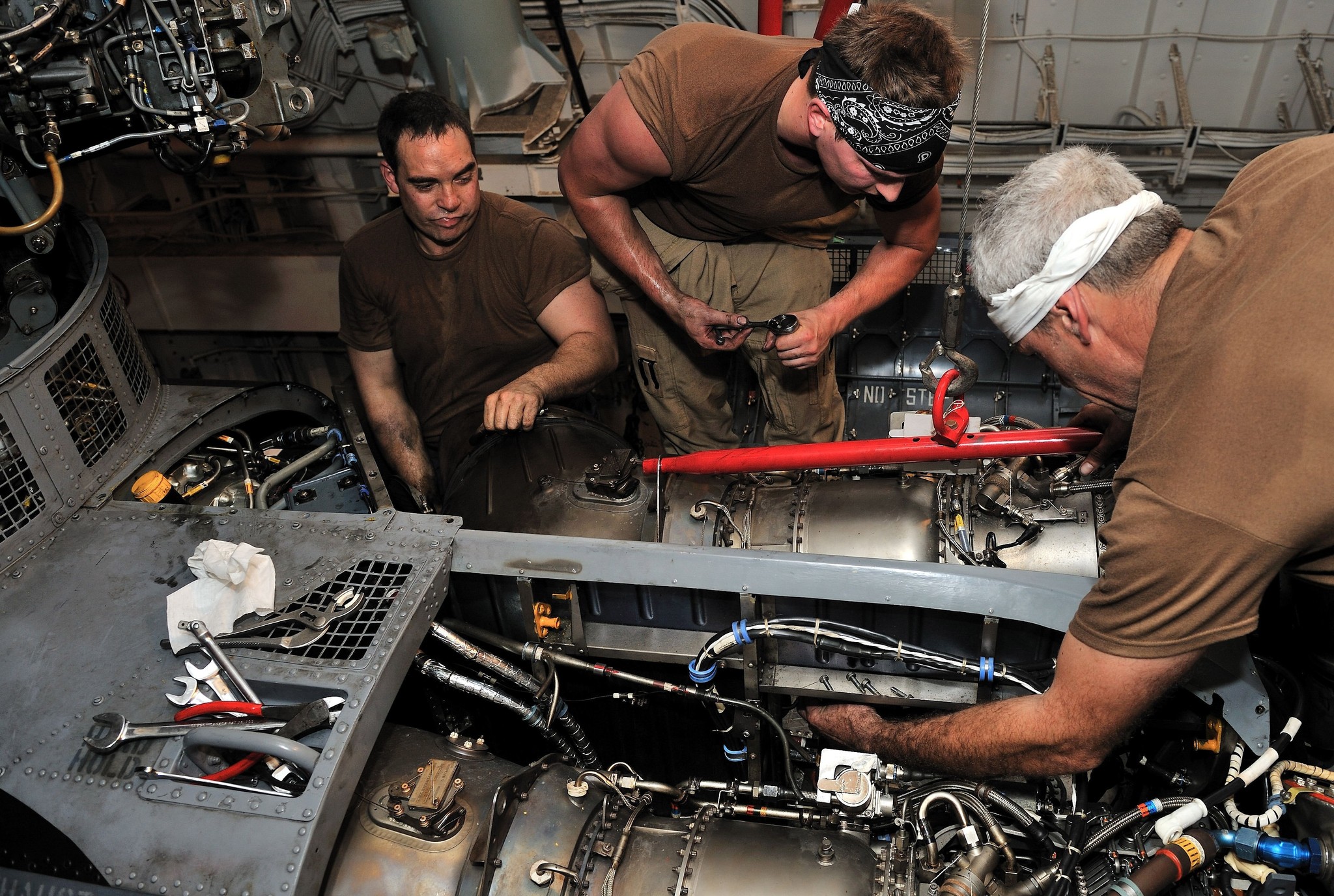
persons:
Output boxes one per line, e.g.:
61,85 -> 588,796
801,128 -> 1333,783
560,2 -> 978,473
333,88 -> 622,511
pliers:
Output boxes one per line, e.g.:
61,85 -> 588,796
176,697 -> 345,781
